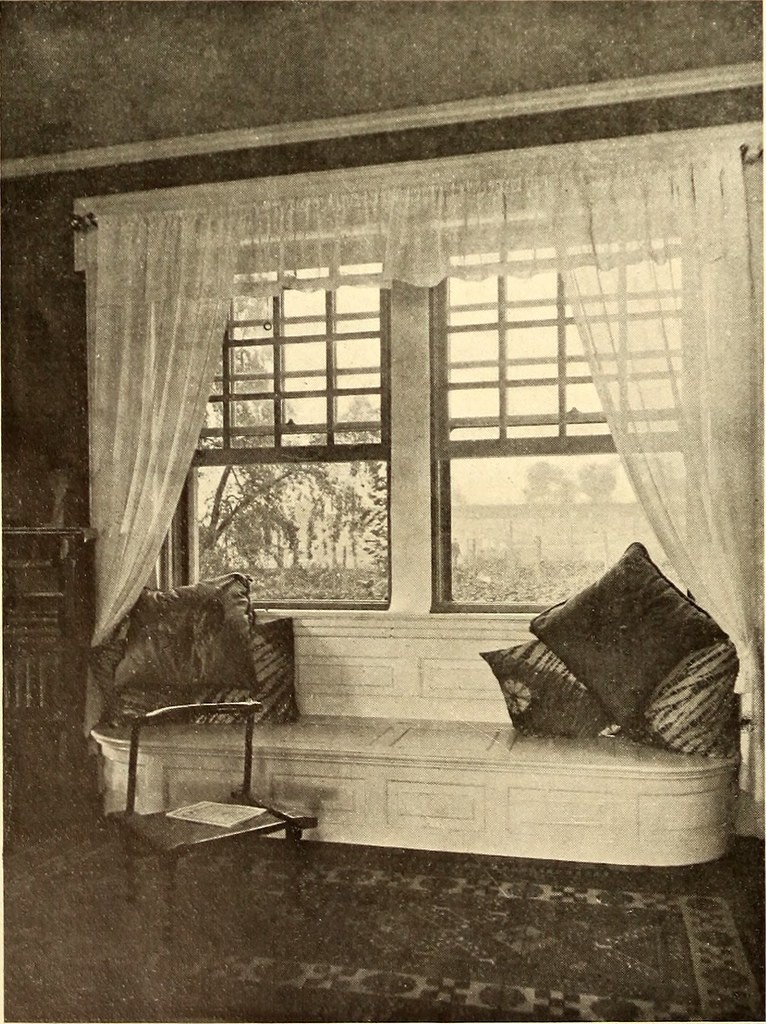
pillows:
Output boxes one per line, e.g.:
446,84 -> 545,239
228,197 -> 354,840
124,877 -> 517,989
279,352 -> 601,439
84,640 -> 191,729
112,573 -> 258,691
633,638 -> 738,760
190,618 -> 302,726
529,542 -> 725,730
480,639 -> 623,740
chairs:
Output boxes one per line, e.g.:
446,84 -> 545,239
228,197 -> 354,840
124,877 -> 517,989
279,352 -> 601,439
107,701 -> 318,939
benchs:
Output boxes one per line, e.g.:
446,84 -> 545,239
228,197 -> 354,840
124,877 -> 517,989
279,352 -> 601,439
90,607 -> 736,868
